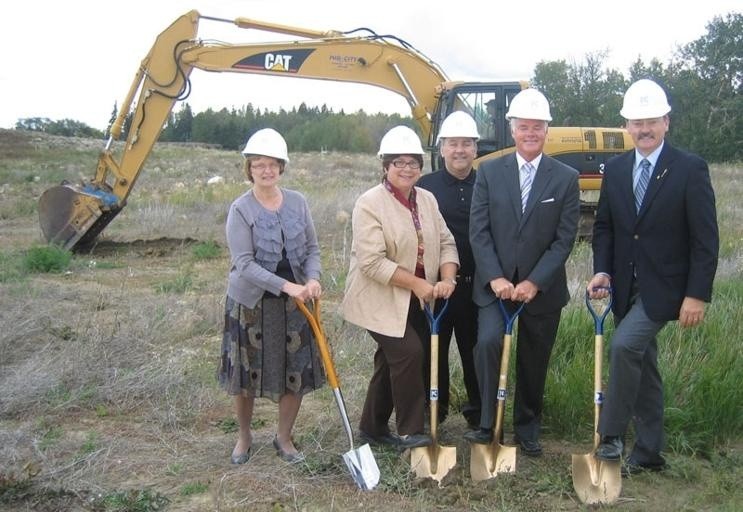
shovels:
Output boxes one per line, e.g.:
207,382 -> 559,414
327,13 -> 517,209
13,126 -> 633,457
411,294 -> 457,487
571,287 -> 622,505
295,293 -> 379,493
470,299 -> 524,487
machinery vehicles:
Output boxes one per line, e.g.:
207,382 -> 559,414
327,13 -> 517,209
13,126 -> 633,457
35,3 -> 629,263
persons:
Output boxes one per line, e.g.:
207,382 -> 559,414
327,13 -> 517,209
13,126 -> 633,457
587,78 -> 719,479
464,86 -> 581,458
216,128 -> 322,465
337,125 -> 460,449
416,109 -> 480,431
483,98 -> 499,138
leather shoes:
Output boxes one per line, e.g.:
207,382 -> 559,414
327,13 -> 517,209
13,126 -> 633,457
595,435 -> 625,460
273,434 -> 304,465
231,430 -> 255,463
622,457 -> 665,477
360,422 -> 543,455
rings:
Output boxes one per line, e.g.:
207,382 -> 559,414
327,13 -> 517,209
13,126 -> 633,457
694,319 -> 698,320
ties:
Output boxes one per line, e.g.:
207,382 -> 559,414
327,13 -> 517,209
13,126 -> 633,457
521,163 -> 532,214
634,159 -> 650,214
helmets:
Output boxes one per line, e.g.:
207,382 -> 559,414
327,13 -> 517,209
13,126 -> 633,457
619,80 -> 672,120
437,111 -> 481,141
378,126 -> 426,155
505,89 -> 553,121
242,128 -> 288,162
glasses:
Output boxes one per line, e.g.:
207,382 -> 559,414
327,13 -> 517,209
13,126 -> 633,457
393,160 -> 420,169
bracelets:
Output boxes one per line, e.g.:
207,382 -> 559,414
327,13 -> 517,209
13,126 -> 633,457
443,278 -> 457,284
